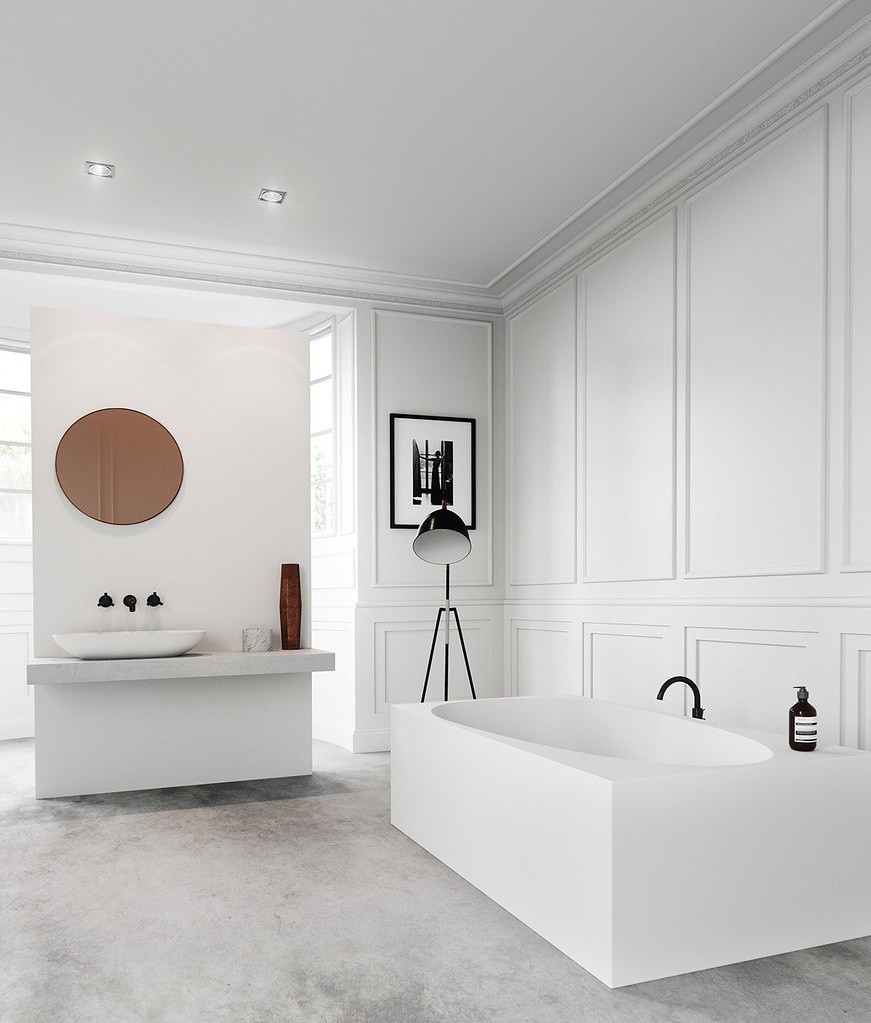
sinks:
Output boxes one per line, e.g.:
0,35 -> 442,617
52,630 -> 207,659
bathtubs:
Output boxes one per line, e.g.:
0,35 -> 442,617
389,692 -> 871,990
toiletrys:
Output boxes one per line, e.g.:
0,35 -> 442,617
789,686 -> 818,751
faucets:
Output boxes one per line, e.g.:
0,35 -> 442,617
123,594 -> 137,612
656,676 -> 706,720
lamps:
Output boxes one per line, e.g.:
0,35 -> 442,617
412,496 -> 477,703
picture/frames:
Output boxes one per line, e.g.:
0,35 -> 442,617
389,413 -> 477,531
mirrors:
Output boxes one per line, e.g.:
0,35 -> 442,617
55,407 -> 184,525
